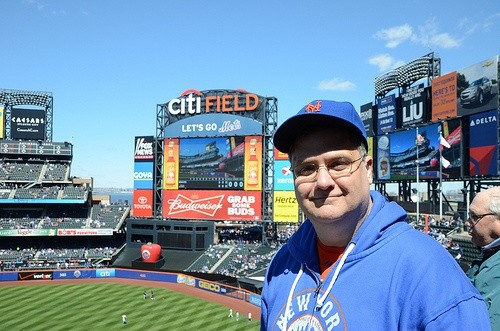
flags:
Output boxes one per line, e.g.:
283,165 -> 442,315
440,136 -> 451,149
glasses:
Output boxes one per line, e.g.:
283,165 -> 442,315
467,212 -> 494,220
290,153 -> 366,180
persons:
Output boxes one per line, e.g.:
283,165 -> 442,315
260,99 -> 492,331
465,187 -> 500,331
410,216 -> 471,260
144,290 -> 153,299
122,314 -> 127,325
229,308 -> 252,322
199,229 -> 293,278
0,161 -> 125,268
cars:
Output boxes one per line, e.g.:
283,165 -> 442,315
460,77 -> 492,107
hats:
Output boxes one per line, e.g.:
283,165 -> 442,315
274,100 -> 369,152
